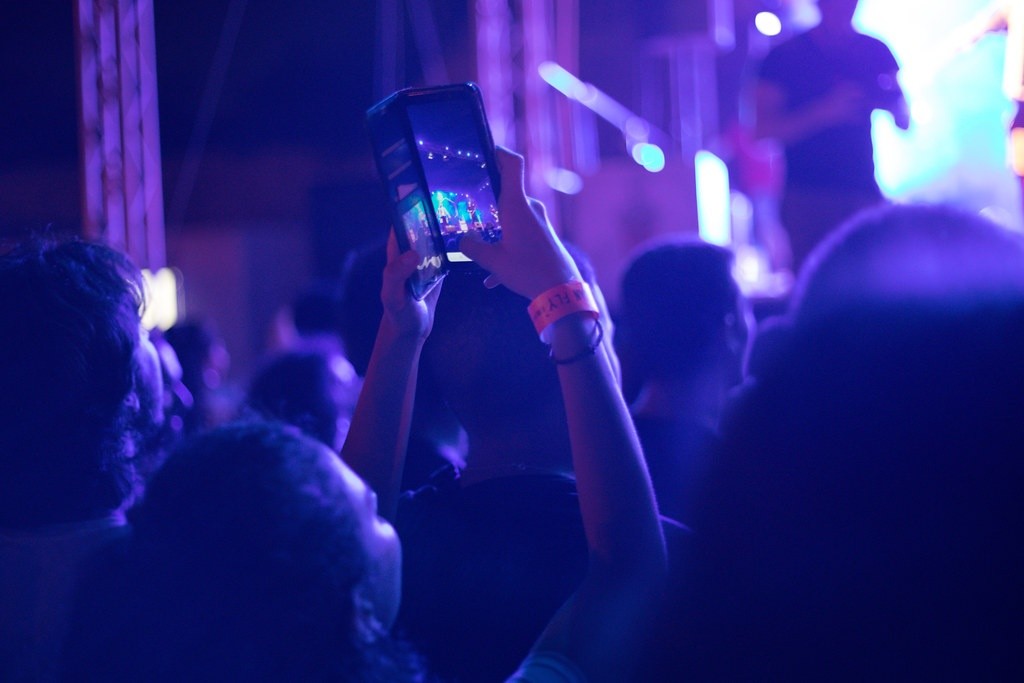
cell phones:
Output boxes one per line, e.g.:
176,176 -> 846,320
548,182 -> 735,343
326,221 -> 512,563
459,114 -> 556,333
401,82 -> 504,281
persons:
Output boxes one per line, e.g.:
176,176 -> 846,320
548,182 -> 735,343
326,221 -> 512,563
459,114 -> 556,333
489,203 -> 499,228
1,1 -> 1024,683
437,205 -> 451,232
467,201 -> 479,224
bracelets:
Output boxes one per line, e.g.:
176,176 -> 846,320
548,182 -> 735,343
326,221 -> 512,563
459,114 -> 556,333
527,280 -> 600,343
548,319 -> 604,364
